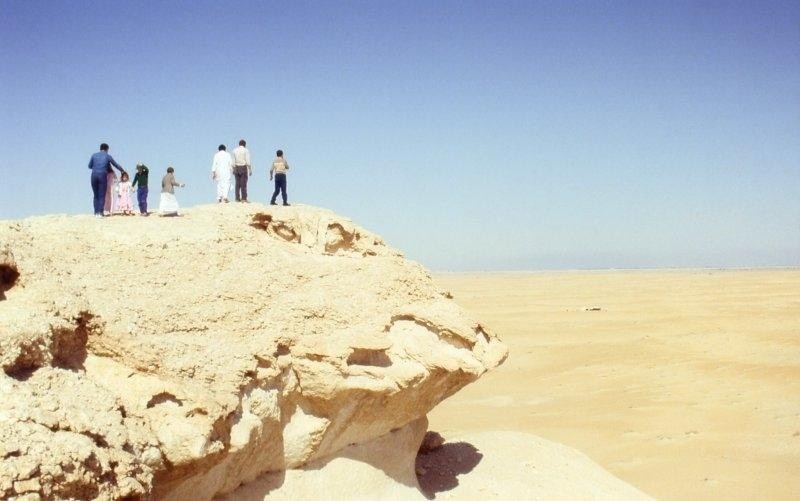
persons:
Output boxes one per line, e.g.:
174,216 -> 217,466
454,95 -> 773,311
210,144 -> 232,203
88,143 -> 129,219
233,139 -> 254,204
156,167 -> 187,218
268,150 -> 292,207
102,163 -> 116,216
117,173 -> 136,217
132,161 -> 149,217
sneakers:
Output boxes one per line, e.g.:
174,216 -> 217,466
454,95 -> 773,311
270,202 -> 290,206
